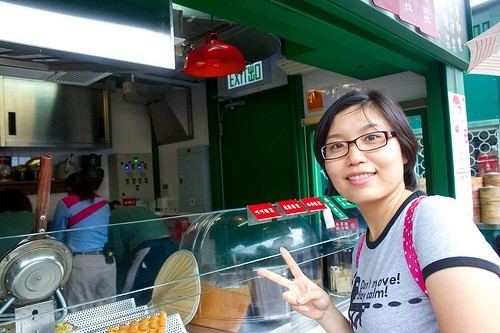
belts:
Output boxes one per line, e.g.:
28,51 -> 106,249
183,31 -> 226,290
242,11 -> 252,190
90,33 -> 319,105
73,251 -> 104,255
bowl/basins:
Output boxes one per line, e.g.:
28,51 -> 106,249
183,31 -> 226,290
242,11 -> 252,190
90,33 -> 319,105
12,165 -> 40,180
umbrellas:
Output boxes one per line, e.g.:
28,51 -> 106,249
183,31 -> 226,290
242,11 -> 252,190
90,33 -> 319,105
463,22 -> 500,76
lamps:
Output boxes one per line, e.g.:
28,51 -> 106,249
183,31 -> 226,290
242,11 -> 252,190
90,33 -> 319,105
184,15 -> 246,77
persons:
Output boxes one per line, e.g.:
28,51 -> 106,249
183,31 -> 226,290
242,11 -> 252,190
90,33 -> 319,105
107,199 -> 178,307
256,87 -> 500,333
0,189 -> 65,322
53,170 -> 117,314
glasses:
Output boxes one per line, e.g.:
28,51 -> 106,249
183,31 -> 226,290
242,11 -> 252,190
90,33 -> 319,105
320,131 -> 397,160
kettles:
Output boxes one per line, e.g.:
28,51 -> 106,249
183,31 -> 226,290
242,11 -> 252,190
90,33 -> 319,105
55,152 -> 78,179
